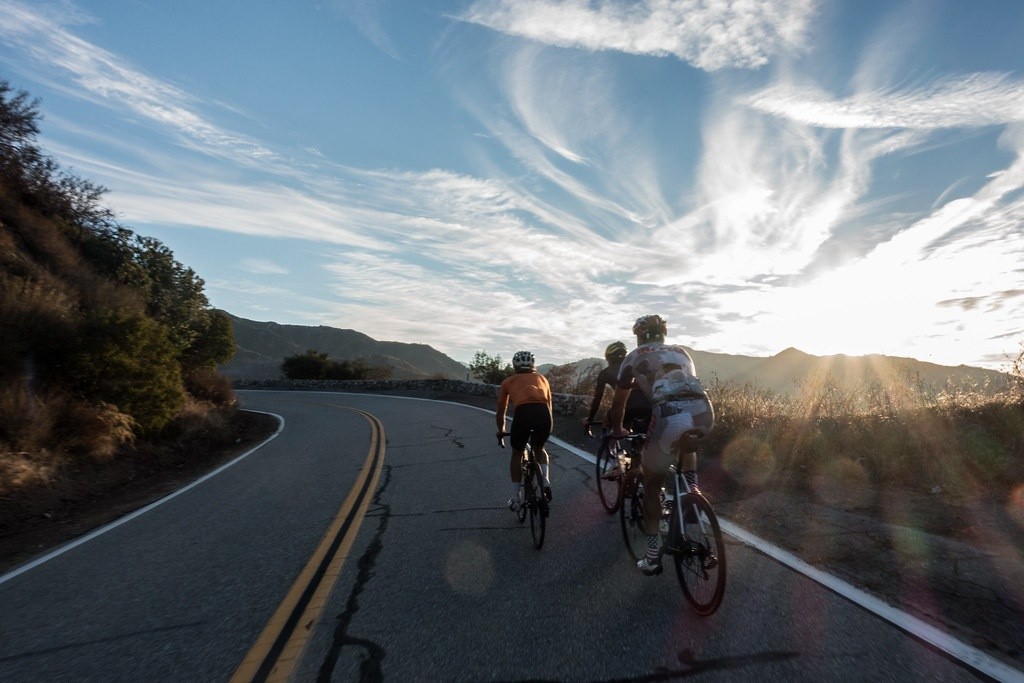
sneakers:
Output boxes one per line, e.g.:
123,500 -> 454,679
637,557 -> 663,574
604,463 -> 625,479
684,482 -> 702,524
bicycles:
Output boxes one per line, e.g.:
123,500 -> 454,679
584,419 -> 728,617
498,428 -> 552,551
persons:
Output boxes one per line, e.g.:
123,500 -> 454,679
611,314 -> 715,575
495,351 -> 552,512
583,341 -> 648,478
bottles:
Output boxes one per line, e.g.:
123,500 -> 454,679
615,450 -> 644,496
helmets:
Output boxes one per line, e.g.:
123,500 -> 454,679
606,341 -> 626,361
513,351 -> 535,370
633,315 -> 667,338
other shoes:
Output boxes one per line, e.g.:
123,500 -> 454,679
508,497 -> 521,512
542,487 -> 552,502
